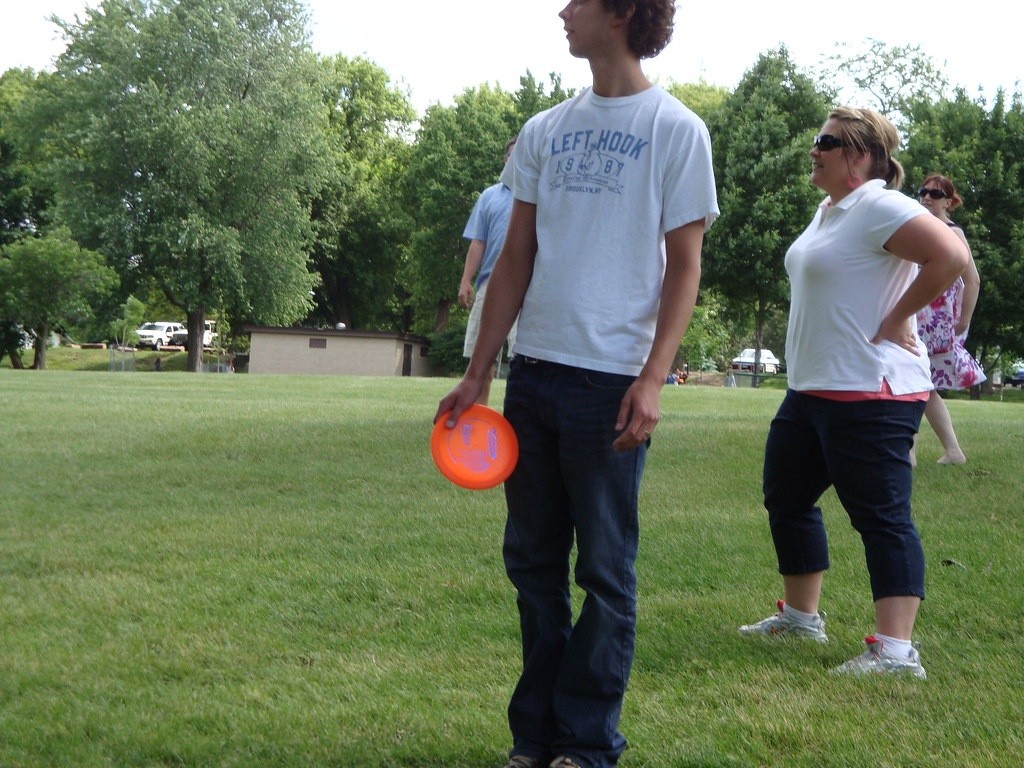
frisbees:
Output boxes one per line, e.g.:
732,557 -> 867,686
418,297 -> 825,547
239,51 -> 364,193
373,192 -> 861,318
429,403 -> 519,490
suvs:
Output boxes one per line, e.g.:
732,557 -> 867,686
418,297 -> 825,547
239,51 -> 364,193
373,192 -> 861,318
136,322 -> 186,351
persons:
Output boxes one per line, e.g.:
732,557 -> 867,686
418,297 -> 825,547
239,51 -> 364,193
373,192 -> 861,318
432,1 -> 719,768
724,367 -> 736,388
668,368 -> 687,384
909,175 -> 987,466
738,107 -> 968,676
458,138 -> 521,406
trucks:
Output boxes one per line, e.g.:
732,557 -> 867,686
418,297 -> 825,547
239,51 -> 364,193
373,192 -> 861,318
167,320 -> 217,350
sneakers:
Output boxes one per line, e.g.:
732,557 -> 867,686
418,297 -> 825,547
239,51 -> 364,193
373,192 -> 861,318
828,635 -> 928,682
737,599 -> 830,645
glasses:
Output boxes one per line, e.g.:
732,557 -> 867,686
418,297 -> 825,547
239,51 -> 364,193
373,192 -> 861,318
917,187 -> 947,199
813,134 -> 849,152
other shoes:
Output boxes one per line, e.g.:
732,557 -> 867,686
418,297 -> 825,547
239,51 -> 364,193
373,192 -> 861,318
548,756 -> 582,768
504,756 -> 547,768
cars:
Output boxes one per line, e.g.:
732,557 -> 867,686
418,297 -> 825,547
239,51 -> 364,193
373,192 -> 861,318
731,349 -> 781,374
1002,362 -> 1024,388
